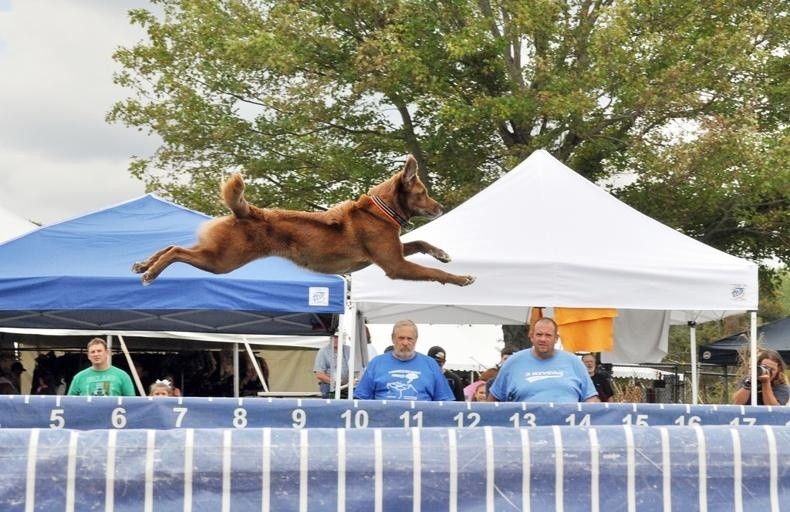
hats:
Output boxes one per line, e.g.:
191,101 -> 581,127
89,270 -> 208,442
428,346 -> 446,362
11,362 -> 26,371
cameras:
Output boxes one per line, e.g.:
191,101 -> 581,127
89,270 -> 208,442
757,363 -> 773,376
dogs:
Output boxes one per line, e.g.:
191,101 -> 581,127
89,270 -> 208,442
132,154 -> 478,287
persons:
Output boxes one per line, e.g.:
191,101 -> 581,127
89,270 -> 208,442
731,350 -> 790,405
37,373 -> 57,394
314,330 -> 350,399
488,317 -> 601,402
355,320 -> 456,401
581,354 -> 614,402
428,346 -> 463,401
0,362 -> 26,395
67,337 -> 135,396
129,348 -> 269,398
472,347 -> 518,401
